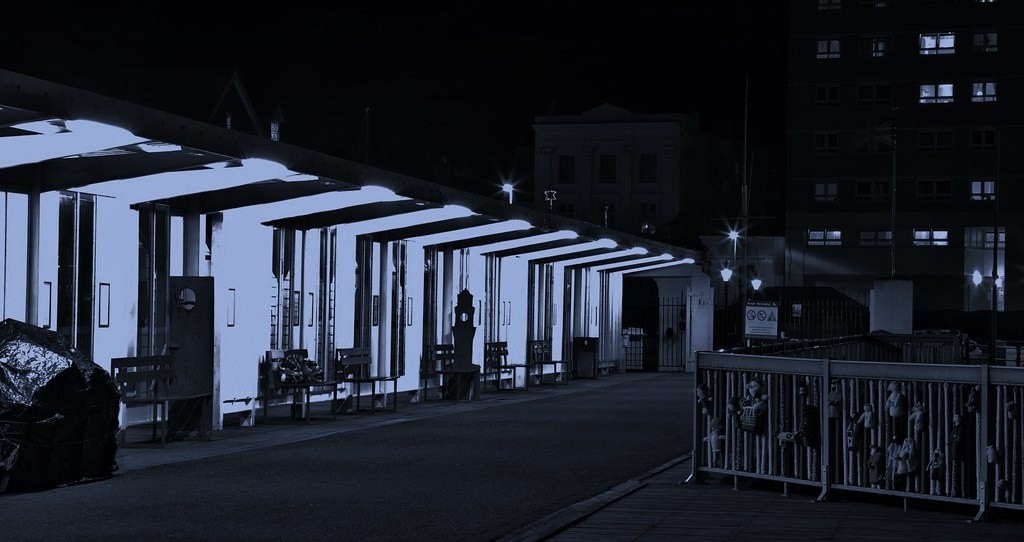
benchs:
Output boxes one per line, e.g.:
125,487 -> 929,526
530,340 -> 570,385
111,356 -> 212,446
424,344 -> 480,402
484,342 -> 530,393
337,348 -> 400,415
266,350 -> 338,424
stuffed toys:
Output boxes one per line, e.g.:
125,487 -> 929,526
926,447 -> 944,496
884,380 -> 905,417
908,399 -> 924,439
846,411 -> 857,450
696,383 -> 713,414
738,377 -> 769,433
897,438 -> 919,493
947,413 -> 966,498
857,404 -> 873,429
703,417 -> 724,462
883,440 -> 901,491
827,380 -> 842,417
728,396 -> 740,429
797,374 -> 821,482
867,444 -> 883,489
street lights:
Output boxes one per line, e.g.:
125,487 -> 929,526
719,266 -> 734,347
